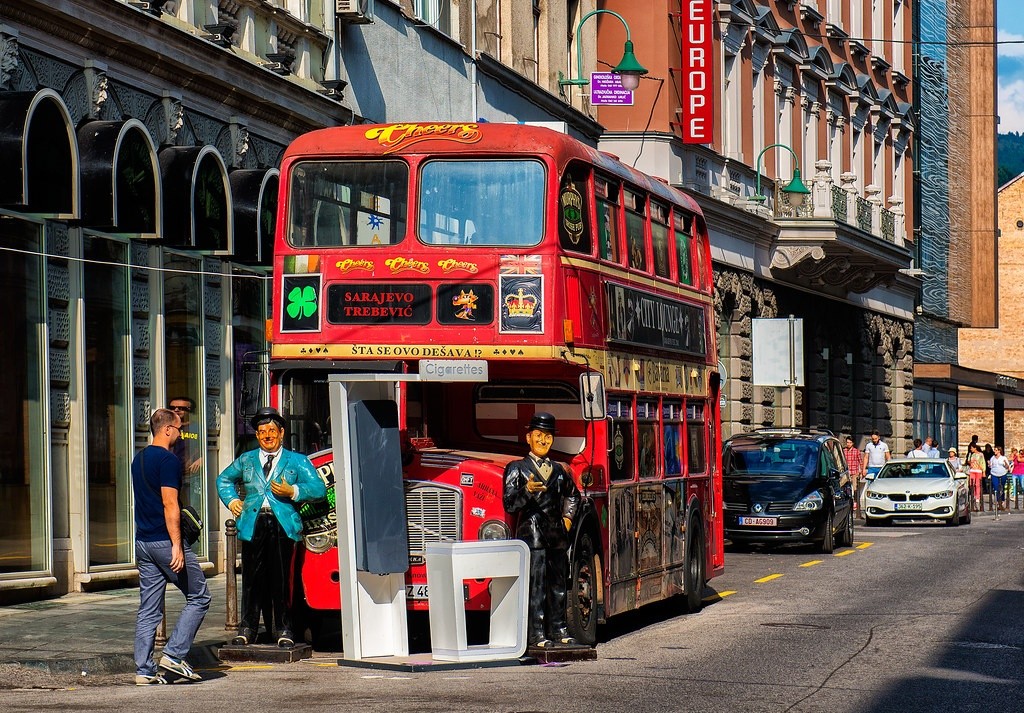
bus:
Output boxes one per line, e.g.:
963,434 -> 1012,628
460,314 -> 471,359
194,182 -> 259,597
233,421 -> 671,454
240,120 -> 725,645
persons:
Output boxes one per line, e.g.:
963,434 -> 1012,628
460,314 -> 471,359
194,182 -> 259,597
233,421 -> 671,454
92,72 -> 110,114
236,126 -> 251,164
0,42 -> 22,84
987,445 -> 1011,511
968,444 -> 986,512
130,409 -> 212,686
1008,447 -> 1024,508
963,435 -> 983,466
984,443 -> 997,477
215,407 -> 326,649
946,446 -> 963,474
905,437 -> 940,473
170,398 -> 203,558
501,411 -> 582,647
170,100 -> 187,142
842,429 -> 892,520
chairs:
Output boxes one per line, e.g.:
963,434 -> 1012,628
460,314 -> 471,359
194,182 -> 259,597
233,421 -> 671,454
931,466 -> 946,474
895,469 -> 911,476
772,450 -> 803,472
734,450 -> 770,472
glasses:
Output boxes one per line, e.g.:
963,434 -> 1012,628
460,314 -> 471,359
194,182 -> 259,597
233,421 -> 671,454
169,405 -> 192,411
167,425 -> 182,434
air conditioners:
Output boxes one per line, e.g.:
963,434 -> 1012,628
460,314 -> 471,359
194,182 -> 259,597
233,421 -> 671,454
336,0 -> 375,25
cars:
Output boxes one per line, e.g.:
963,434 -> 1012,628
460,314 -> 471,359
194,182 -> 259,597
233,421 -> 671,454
864,458 -> 971,526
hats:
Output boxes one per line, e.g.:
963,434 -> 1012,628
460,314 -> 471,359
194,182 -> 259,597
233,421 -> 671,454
948,447 -> 957,455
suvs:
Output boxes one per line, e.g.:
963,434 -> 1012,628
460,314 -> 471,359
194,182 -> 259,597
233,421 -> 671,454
722,427 -> 854,554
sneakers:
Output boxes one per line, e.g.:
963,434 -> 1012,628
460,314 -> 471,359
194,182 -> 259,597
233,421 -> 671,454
159,654 -> 202,682
135,673 -> 174,686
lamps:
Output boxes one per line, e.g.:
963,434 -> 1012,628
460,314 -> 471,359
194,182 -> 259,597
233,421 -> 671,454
201,24 -> 237,48
316,79 -> 348,101
558,10 -> 649,95
263,54 -> 296,76
128,0 -> 168,18
749,145 -> 811,206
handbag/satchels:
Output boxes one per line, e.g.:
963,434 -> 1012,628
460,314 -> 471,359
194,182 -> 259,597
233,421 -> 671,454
180,506 -> 203,546
